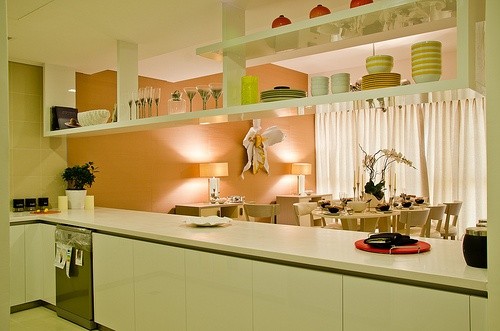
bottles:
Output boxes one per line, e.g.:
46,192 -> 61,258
167,92 -> 186,114
461,218 -> 487,269
272,14 -> 291,28
309,4 -> 331,18
350,0 -> 373,8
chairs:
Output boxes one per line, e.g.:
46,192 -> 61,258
243,202 -> 280,224
293,200 -> 463,240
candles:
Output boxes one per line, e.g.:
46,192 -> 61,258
394,172 -> 396,189
358,165 -> 360,182
362,173 -> 364,190
384,169 -> 386,187
354,170 -> 356,187
390,167 -> 392,185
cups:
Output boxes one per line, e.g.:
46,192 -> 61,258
84,195 -> 95,208
241,76 -> 258,105
58,195 -> 69,211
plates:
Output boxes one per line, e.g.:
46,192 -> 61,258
259,88 -> 306,102
183,215 -> 233,227
361,73 -> 400,89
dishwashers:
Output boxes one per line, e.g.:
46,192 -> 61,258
53,223 -> 98,330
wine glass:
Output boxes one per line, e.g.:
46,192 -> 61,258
206,86 -> 212,101
183,87 -> 197,111
209,83 -> 223,109
195,85 -> 209,110
126,85 -> 160,120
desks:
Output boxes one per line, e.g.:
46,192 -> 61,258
312,203 -> 439,232
175,203 -> 256,222
276,194 -> 334,225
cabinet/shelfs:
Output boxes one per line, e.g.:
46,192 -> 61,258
195,0 -> 485,125
91,231 -> 489,331
9,223 -> 42,306
43,223 -> 56,306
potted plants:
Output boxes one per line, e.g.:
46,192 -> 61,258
60,159 -> 101,210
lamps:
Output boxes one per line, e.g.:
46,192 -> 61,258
200,162 -> 228,204
292,162 -> 312,195
349,40 -> 410,113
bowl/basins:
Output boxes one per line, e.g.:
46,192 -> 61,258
310,72 -> 349,96
216,198 -> 227,204
366,39 -> 442,85
77,110 -> 110,126
321,195 -> 424,215
